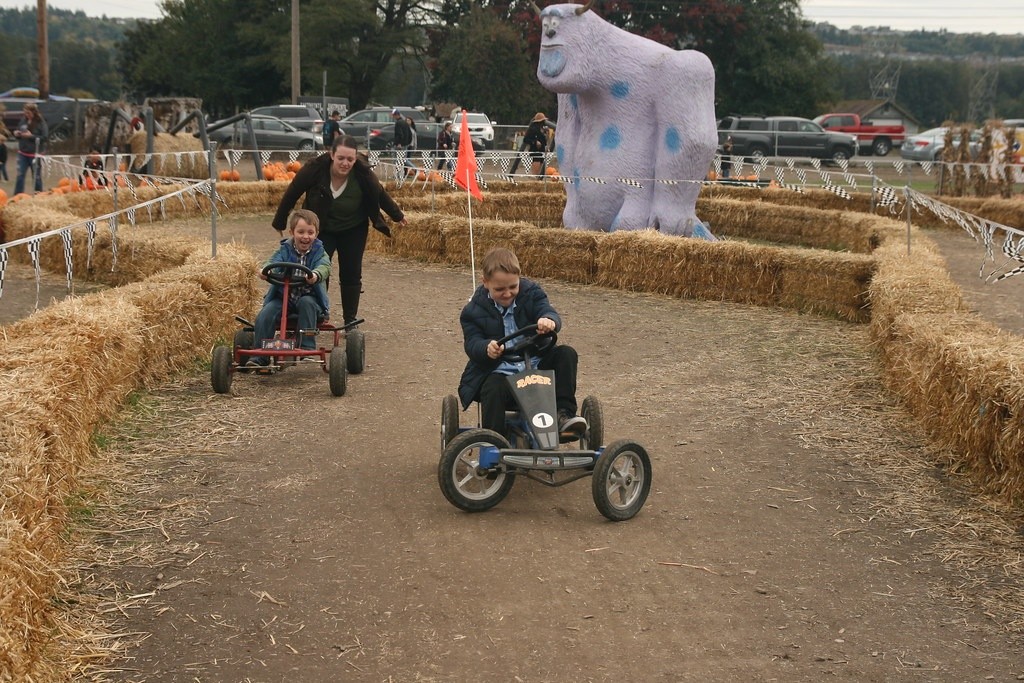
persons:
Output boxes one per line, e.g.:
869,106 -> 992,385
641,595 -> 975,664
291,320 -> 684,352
405,116 -> 418,152
322,110 -> 341,150
11,102 -> 48,195
458,246 -> 587,445
436,123 -> 455,171
507,112 -> 557,180
271,135 -> 408,339
720,143 -> 732,177
390,109 -> 412,180
78,143 -> 108,187
245,209 -> 332,365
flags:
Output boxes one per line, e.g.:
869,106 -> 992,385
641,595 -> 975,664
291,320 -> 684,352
454,110 -> 483,202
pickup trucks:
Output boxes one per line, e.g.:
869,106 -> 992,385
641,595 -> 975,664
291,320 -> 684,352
811,114 -> 905,156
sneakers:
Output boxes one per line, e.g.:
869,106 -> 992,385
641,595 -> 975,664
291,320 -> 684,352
557,406 -> 587,444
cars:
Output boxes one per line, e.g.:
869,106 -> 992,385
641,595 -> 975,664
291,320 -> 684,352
901,126 -> 986,163
0,98 -> 42,130
207,114 -> 324,151
365,120 -> 486,158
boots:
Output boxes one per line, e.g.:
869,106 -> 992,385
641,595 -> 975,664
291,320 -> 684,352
340,283 -> 357,337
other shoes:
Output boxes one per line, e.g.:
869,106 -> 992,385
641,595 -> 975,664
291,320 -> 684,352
246,355 -> 265,367
298,336 -> 316,349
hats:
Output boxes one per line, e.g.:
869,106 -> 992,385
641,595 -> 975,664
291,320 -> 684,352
533,113 -> 548,122
391,108 -> 399,114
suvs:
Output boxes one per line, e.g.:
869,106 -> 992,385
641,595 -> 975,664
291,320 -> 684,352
717,115 -> 858,164
18,99 -> 110,143
245,104 -> 325,134
336,105 -> 427,143
451,112 -> 495,149
